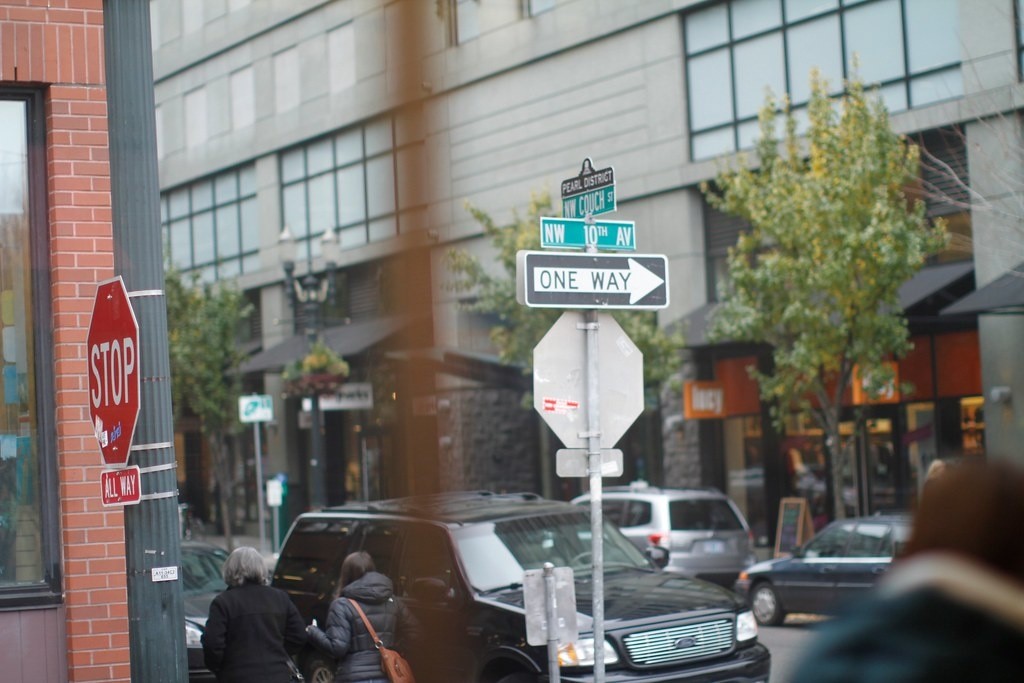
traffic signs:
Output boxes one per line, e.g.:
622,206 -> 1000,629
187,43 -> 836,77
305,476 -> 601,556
515,249 -> 670,312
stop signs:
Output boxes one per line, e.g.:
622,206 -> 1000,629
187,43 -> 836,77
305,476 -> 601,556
86,275 -> 142,467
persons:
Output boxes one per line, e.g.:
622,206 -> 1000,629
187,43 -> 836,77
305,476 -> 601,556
305,550 -> 423,683
200,546 -> 308,683
777,450 -> 1024,683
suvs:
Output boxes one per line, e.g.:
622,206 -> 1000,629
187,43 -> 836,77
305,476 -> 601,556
270,486 -> 773,683
569,486 -> 757,592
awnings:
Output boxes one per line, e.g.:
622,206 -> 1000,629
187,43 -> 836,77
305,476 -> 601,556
942,261 -> 1024,315
650,258 -> 976,348
225,312 -> 406,377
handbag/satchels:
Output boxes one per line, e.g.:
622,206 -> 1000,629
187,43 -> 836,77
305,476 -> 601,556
290,674 -> 306,683
379,645 -> 415,683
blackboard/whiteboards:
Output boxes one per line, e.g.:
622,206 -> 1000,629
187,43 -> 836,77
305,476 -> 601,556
775,498 -> 803,556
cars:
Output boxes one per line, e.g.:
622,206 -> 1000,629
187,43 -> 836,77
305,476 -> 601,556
179,539 -> 272,683
728,510 -> 914,627
